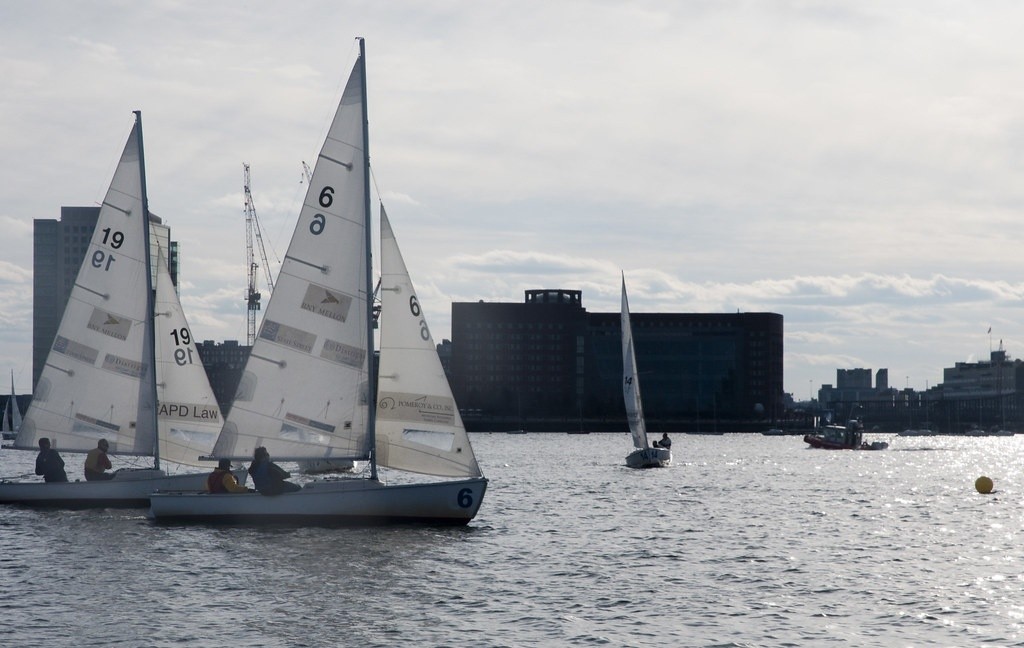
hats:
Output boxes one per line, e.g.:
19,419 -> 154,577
218,457 -> 234,467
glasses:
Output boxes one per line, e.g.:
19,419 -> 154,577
103,443 -> 109,447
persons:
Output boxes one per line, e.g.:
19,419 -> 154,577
80,438 -> 114,482
33,437 -> 69,482
205,458 -> 250,494
658,431 -> 672,449
248,446 -> 303,497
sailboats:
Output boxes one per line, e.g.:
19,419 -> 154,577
0,109 -> 249,509
295,277 -> 383,475
620,271 -> 672,468
2,368 -> 24,440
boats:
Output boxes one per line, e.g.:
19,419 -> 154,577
762,425 -> 1016,436
805,421 -> 887,450
144,37 -> 487,529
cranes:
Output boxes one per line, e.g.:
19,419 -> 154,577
238,157 -> 312,345
